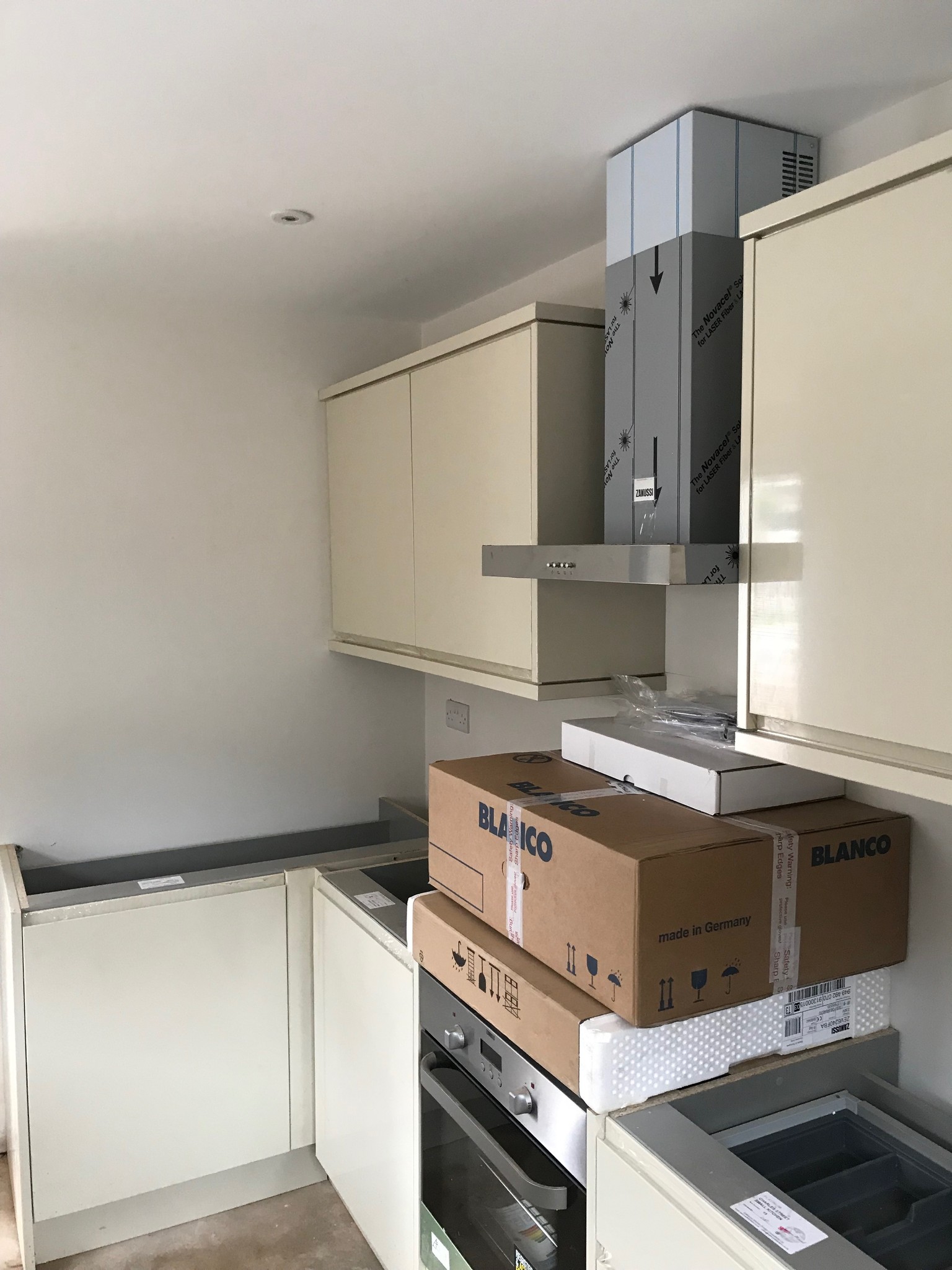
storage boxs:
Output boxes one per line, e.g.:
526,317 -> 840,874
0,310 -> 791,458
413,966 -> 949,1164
405,890 -> 892,1116
428,748 -> 913,1027
562,716 -> 846,815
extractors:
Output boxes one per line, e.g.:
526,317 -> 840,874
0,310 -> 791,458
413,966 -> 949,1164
479,109 -> 821,584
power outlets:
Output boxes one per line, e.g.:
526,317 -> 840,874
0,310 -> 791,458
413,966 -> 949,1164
446,699 -> 469,733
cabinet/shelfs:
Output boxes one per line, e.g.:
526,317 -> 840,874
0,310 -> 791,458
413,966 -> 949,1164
0,882 -> 414,1270
734,132 -> 952,807
594,1137 -> 789,1270
318,302 -> 667,701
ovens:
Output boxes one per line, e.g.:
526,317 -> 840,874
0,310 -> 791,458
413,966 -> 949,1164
417,968 -> 586,1270
